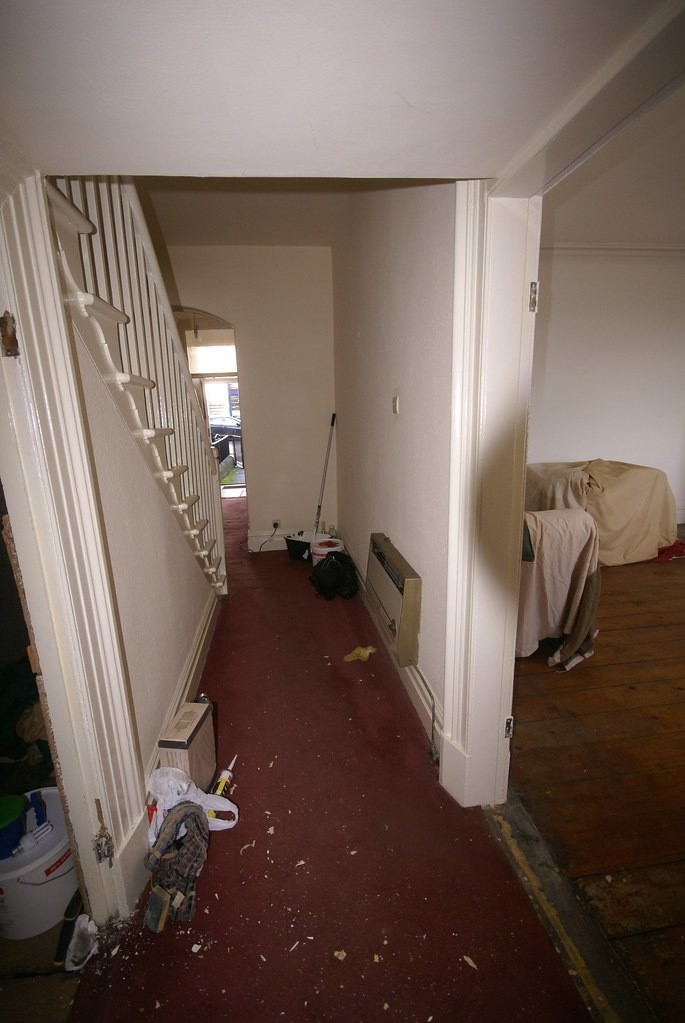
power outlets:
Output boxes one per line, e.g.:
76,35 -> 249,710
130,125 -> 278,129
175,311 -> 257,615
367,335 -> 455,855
272,519 -> 280,528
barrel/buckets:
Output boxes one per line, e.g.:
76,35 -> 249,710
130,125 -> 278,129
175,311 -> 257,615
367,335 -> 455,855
310,539 -> 344,567
1,786 -> 80,943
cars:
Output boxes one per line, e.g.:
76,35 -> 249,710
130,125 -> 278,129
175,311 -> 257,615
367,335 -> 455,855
210,416 -> 242,443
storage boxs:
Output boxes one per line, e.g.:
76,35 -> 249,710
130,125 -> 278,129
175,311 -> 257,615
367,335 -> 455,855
283,530 -> 335,563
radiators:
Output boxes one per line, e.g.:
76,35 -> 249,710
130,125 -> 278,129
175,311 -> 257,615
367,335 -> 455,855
359,532 -> 424,671
